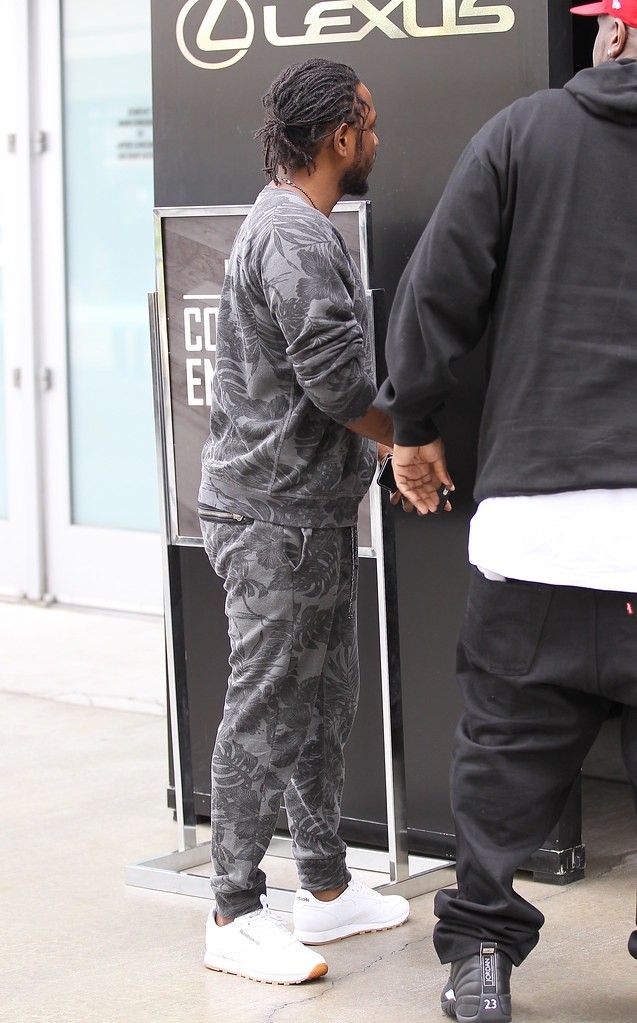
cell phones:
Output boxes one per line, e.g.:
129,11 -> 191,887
377,455 -> 450,514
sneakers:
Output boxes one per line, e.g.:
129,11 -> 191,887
292,876 -> 410,947
204,894 -> 328,985
440,942 -> 511,1023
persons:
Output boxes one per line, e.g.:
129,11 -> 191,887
385,0 -> 637,1023
196,58 -> 451,986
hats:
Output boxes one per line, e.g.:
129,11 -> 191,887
569,0 -> 636,28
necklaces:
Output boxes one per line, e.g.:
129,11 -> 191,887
275,174 -> 321,211
